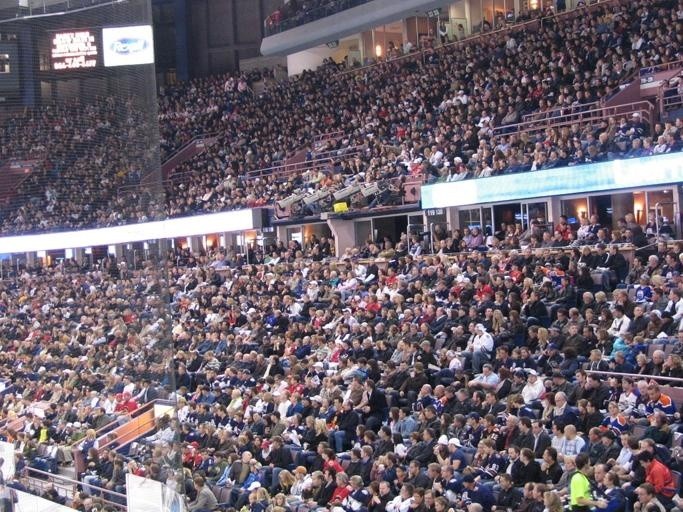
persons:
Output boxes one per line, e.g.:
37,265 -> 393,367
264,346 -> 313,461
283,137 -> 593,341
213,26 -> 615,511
0,1 -> 683,236
0,214 -> 682,512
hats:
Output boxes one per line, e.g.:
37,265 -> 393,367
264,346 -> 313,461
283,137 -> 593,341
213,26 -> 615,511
459,476 -> 474,483
438,434 -> 462,447
62,419 -> 81,430
462,411 -> 480,422
312,294 -> 361,360
526,369 -> 538,376
